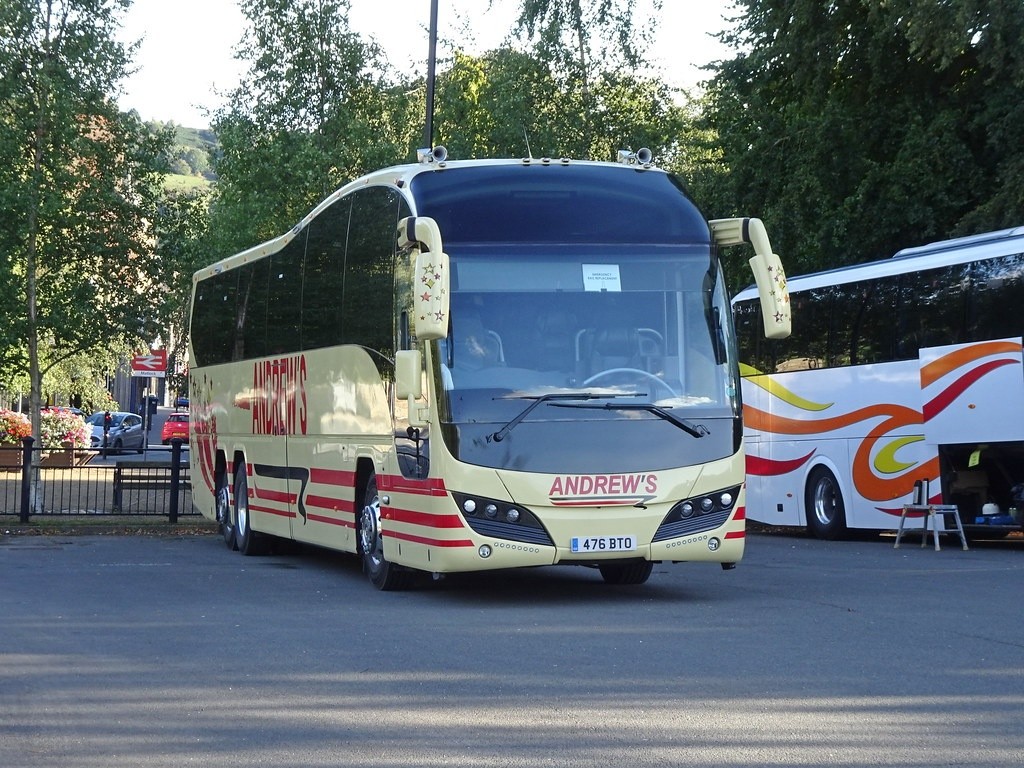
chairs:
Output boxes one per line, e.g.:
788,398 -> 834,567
589,322 -> 649,380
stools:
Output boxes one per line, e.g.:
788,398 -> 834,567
893,504 -> 970,552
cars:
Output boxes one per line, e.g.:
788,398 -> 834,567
173,395 -> 189,408
40,405 -> 89,420
161,412 -> 190,445
83,411 -> 148,456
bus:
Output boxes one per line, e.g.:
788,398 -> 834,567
729,223 -> 1024,546
188,144 -> 793,592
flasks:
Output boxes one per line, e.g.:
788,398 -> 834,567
921,478 -> 929,505
913,480 -> 921,505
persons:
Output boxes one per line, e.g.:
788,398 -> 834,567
450,303 -> 499,366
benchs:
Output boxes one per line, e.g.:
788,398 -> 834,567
113,460 -> 192,515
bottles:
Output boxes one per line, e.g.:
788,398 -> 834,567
441,363 -> 455,390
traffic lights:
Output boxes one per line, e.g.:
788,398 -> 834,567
103,412 -> 112,432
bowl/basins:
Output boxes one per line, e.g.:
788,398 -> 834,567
982,504 -> 1000,515
